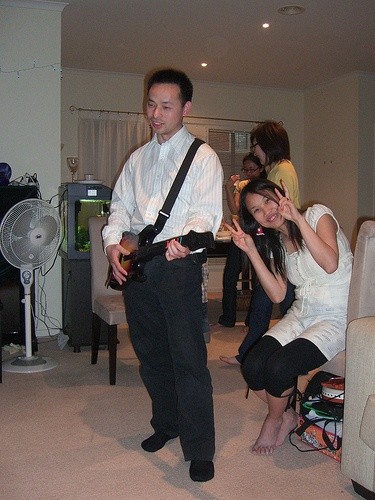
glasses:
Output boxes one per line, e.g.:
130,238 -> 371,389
242,167 -> 259,172
250,143 -> 258,154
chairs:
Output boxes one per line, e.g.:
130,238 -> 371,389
86,215 -> 135,387
289,218 -> 375,412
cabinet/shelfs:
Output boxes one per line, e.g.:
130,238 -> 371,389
62,258 -> 121,351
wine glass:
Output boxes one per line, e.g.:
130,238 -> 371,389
67,156 -> 80,183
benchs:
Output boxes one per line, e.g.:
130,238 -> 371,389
338,312 -> 375,500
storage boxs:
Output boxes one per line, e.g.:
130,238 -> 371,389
295,416 -> 341,463
58,183 -> 113,257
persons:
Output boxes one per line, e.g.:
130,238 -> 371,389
224,179 -> 353,455
102,69 -> 225,482
209,152 -> 267,334
220,120 -> 300,366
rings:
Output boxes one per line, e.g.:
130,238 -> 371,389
287,198 -> 289,200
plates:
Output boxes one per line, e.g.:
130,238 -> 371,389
78,180 -> 103,184
216,231 -> 232,236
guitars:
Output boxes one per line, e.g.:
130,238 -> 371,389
107,228 -> 215,292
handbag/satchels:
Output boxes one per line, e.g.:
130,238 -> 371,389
297,371 -> 348,451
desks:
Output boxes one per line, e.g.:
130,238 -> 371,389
209,238 -> 254,290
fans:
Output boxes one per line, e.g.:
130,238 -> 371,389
0,198 -> 62,375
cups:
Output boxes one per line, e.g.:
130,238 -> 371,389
85,175 -> 93,181
234,175 -> 241,186
